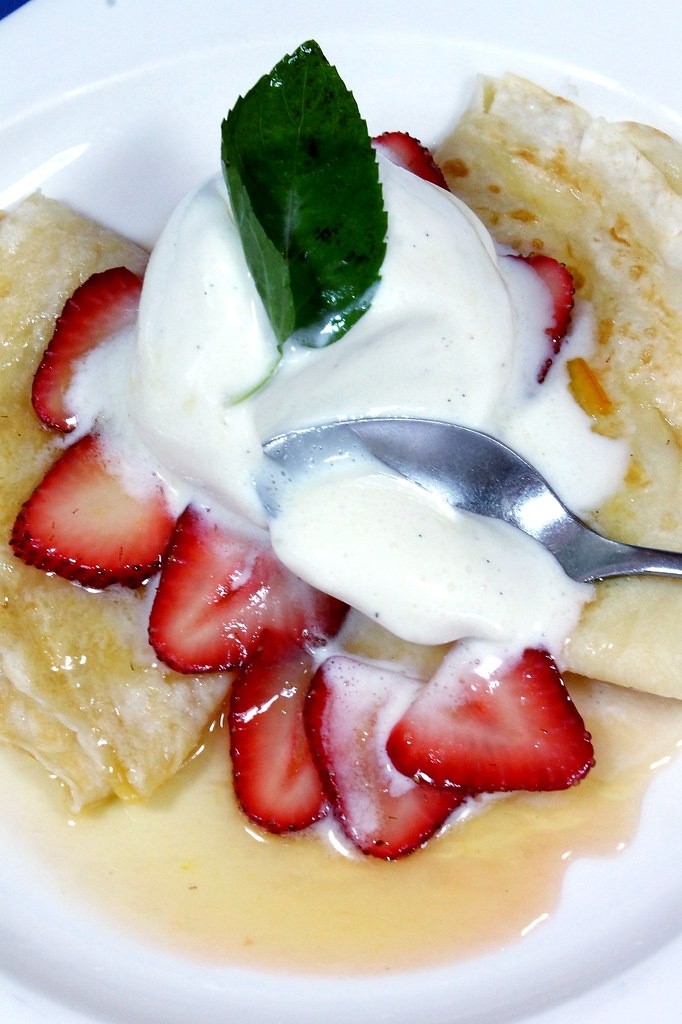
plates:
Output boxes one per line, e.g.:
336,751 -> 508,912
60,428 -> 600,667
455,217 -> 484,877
0,0 -> 682,1018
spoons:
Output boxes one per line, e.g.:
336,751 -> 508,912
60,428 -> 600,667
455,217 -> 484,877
252,415 -> 682,606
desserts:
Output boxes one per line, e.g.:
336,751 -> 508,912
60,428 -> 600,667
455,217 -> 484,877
0,38 -> 682,979
